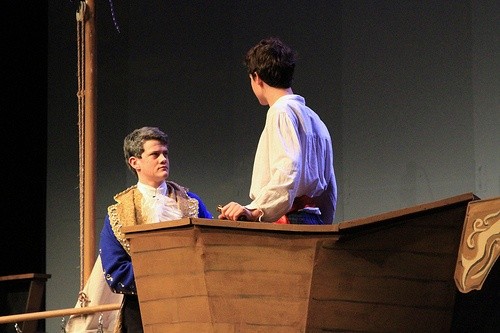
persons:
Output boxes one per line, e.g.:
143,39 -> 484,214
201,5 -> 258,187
98,128 -> 213,333
218,38 -> 338,226
